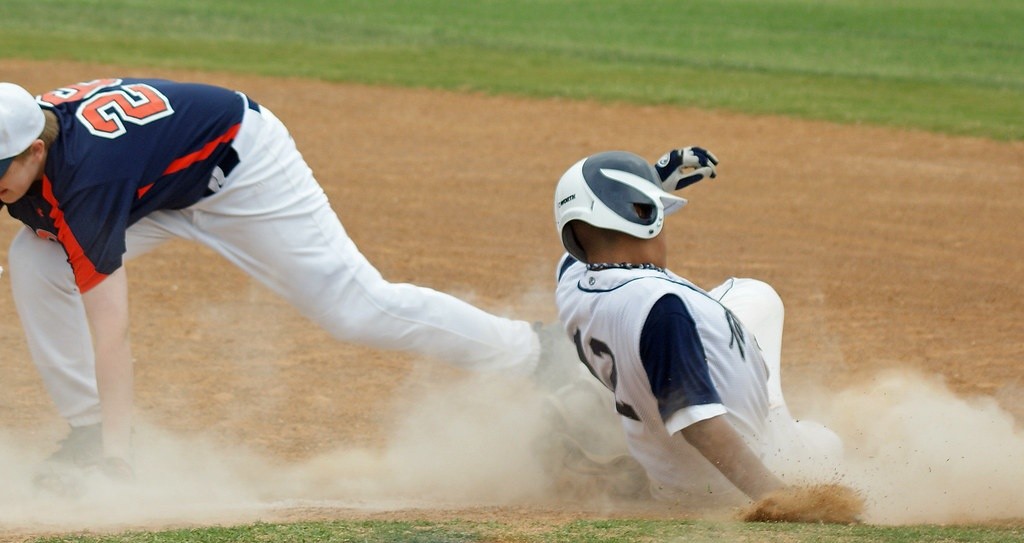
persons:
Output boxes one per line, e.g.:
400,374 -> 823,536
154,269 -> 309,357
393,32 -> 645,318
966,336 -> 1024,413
555,146 -> 858,510
0,76 -> 555,498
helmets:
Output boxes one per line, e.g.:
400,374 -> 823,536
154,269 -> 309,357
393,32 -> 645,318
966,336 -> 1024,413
554,150 -> 689,264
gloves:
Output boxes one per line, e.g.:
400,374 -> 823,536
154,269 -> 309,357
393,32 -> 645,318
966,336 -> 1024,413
654,147 -> 719,191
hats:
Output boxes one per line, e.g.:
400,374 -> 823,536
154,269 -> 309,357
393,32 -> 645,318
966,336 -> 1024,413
0,83 -> 46,179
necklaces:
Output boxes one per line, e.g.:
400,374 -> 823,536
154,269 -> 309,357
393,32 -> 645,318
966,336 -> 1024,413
586,262 -> 668,275
